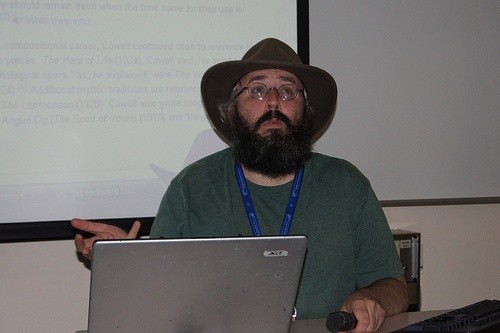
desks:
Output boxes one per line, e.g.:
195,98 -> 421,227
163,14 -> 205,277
288,309 -> 457,332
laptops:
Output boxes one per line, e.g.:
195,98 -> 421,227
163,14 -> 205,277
75,234 -> 308,333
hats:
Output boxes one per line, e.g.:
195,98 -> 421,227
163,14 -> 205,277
200,38 -> 337,147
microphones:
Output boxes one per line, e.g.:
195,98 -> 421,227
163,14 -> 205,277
326,311 -> 357,333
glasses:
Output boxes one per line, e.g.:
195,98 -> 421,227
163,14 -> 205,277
231,81 -> 305,101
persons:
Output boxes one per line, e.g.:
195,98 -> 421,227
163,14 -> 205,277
67,37 -> 412,333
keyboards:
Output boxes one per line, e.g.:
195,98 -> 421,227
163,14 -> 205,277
389,298 -> 500,333
